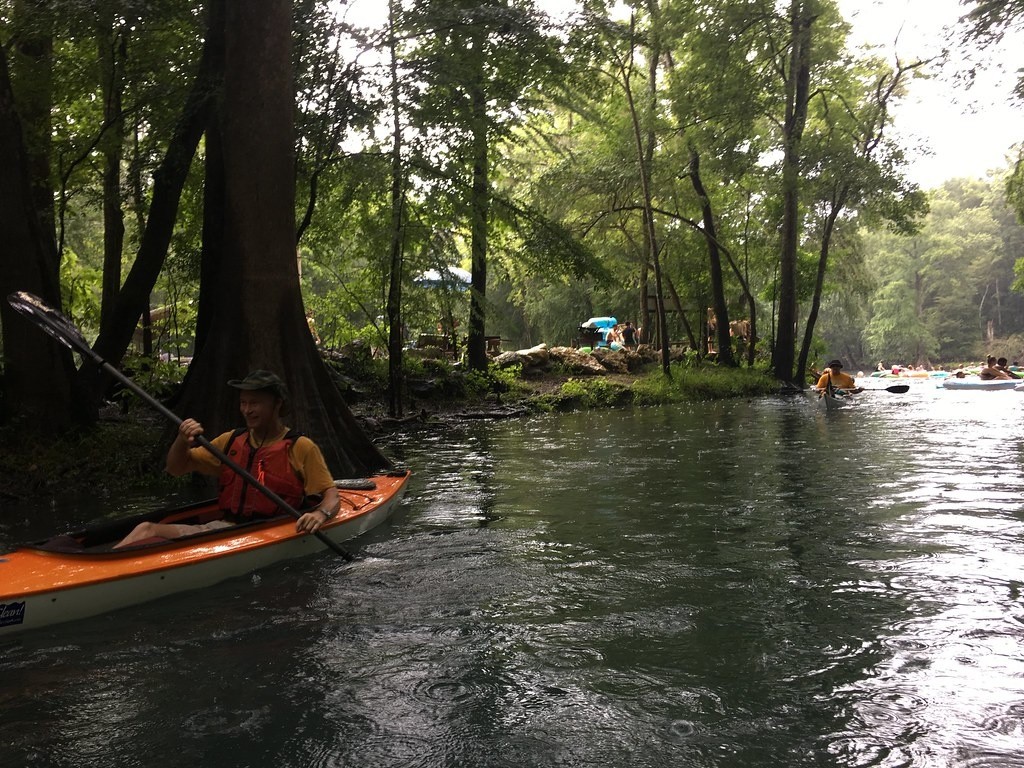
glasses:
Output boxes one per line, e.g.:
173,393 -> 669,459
831,364 -> 840,367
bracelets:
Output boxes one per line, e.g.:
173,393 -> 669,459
317,508 -> 332,520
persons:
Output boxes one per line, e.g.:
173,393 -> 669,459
606,325 -> 620,348
111,369 -> 341,548
730,318 -> 750,354
708,307 -> 717,354
938,354 -> 1024,380
622,322 -> 635,346
892,364 -> 924,374
875,360 -> 885,372
306,308 -> 320,343
815,359 -> 864,398
633,328 -> 641,343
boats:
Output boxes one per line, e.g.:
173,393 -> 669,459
0,470 -> 411,634
824,393 -> 852,410
943,377 -> 1024,389
872,370 -> 1018,378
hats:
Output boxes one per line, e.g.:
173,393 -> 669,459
227,369 -> 294,418
829,360 -> 843,368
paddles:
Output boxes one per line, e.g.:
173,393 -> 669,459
798,385 -> 909,394
6,289 -> 357,565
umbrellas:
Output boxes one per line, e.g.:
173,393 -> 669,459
418,283 -> 468,292
414,265 -> 471,284
